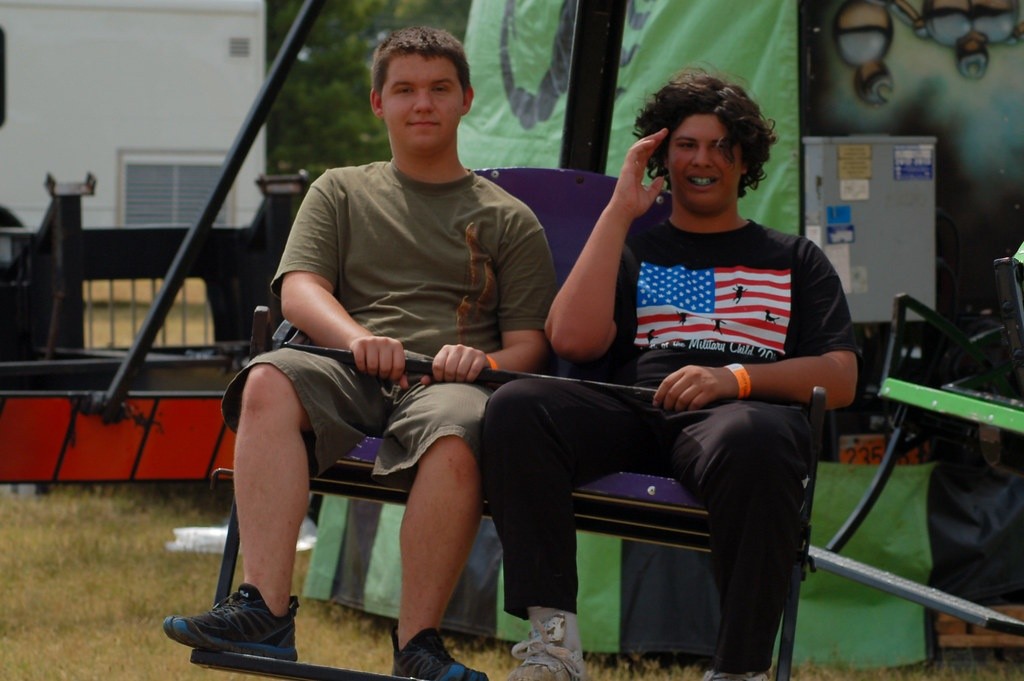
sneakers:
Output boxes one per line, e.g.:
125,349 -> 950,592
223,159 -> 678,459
390,625 -> 489,681
163,583 -> 299,661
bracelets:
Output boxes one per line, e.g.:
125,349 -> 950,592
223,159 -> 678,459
723,363 -> 751,398
485,354 -> 499,371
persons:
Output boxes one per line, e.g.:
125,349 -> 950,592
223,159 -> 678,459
161,25 -> 556,680
477,69 -> 861,681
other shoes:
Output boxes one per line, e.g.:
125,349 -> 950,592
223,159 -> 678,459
510,612 -> 586,681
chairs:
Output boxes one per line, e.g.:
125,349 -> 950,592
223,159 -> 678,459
809,255 -> 1024,634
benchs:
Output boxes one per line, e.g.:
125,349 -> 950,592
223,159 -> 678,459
205,165 -> 826,681
